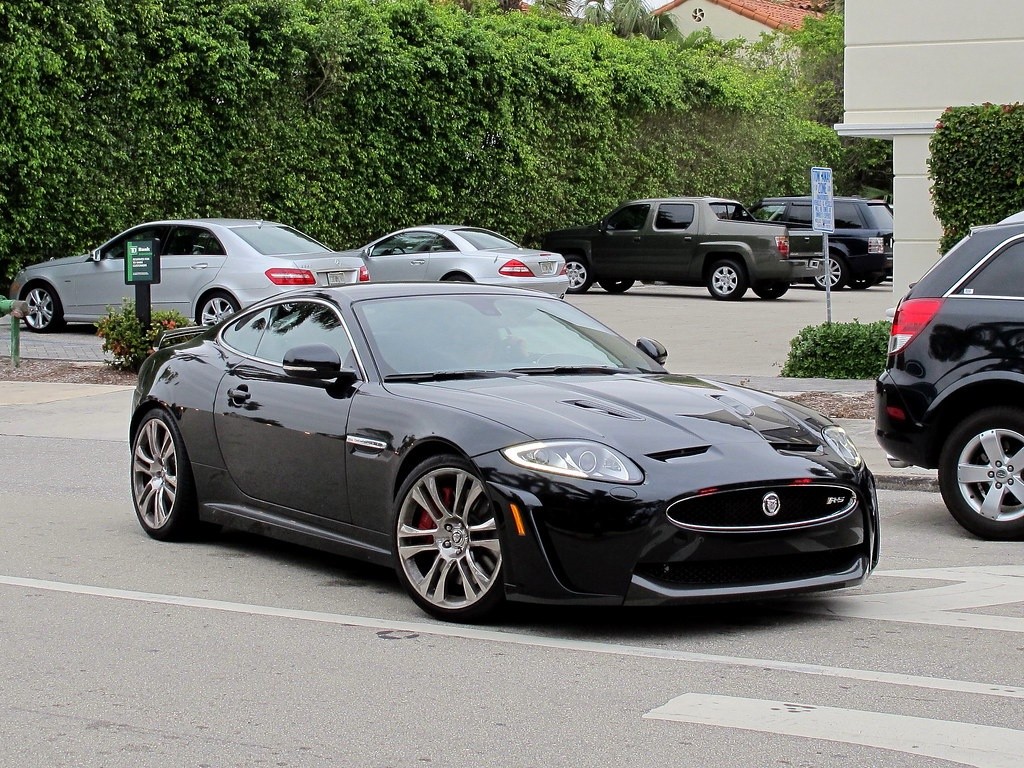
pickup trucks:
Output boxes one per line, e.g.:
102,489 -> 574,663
541,196 -> 825,302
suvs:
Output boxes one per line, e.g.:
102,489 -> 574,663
742,196 -> 893,291
876,210 -> 1024,541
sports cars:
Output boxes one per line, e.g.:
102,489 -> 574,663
129,280 -> 883,624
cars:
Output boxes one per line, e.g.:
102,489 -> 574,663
8,217 -> 371,334
362,224 -> 570,300
